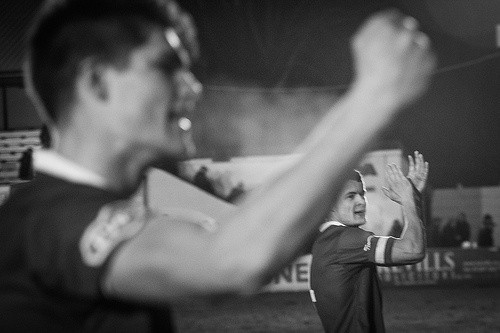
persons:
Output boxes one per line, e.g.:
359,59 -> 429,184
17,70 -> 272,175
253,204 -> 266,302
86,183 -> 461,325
309,150 -> 431,333
153,150 -> 248,203
429,212 -> 496,271
387,220 -> 403,238
0,0 -> 436,333
